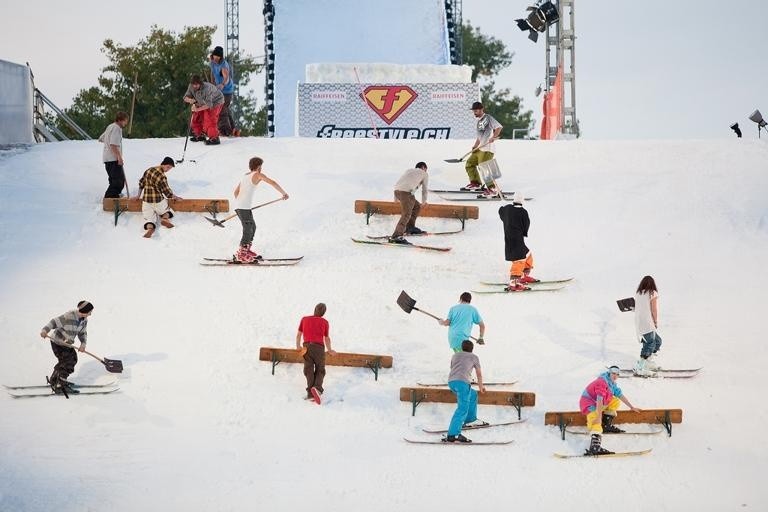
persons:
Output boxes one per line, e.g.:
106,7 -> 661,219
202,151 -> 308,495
40,300 -> 94,395
232,156 -> 290,264
181,74 -> 226,147
97,110 -> 130,197
579,364 -> 641,454
206,45 -> 242,139
497,194 -> 540,293
439,290 -> 486,383
460,101 -> 505,200
296,301 -> 336,405
444,339 -> 489,445
389,162 -> 430,245
633,274 -> 663,379
133,156 -> 182,240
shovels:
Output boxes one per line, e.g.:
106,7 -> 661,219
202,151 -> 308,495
41,334 -> 123,373
205,197 -> 284,227
617,297 -> 635,312
396,290 -> 485,344
444,137 -> 501,163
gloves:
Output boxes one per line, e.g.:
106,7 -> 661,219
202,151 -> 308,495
282,194 -> 290,201
438,316 -> 444,326
475,336 -> 486,346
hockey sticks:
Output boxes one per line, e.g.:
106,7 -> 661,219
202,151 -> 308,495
177,102 -> 195,163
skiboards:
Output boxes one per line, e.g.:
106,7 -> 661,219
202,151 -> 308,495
470,277 -> 576,294
430,191 -> 534,201
605,366 -> 703,378
417,380 -> 518,387
199,256 -> 304,266
554,429 -> 663,458
404,417 -> 530,445
2,382 -> 121,397
352,231 -> 461,251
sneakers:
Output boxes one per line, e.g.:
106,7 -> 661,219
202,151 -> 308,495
204,137 -> 221,145
143,227 -> 155,240
160,217 -> 175,230
189,134 -> 207,142
305,395 -> 315,401
310,386 -> 322,406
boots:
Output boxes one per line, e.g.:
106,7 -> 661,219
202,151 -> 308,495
601,412 -> 627,433
387,234 -> 413,247
463,419 -> 491,426
446,432 -> 473,444
49,373 -> 80,395
505,278 -> 532,292
232,243 -> 263,264
476,186 -> 503,200
645,354 -> 664,370
633,361 -> 656,377
406,225 -> 428,235
460,181 -> 483,192
584,434 -> 616,455
520,272 -> 541,284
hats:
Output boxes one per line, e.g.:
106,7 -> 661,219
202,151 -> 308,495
213,45 -> 224,57
76,300 -> 94,314
469,101 -> 484,111
160,155 -> 176,168
512,194 -> 525,205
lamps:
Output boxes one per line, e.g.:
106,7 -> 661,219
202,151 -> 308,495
509,0 -> 561,43
748,108 -> 768,139
729,121 -> 743,138
